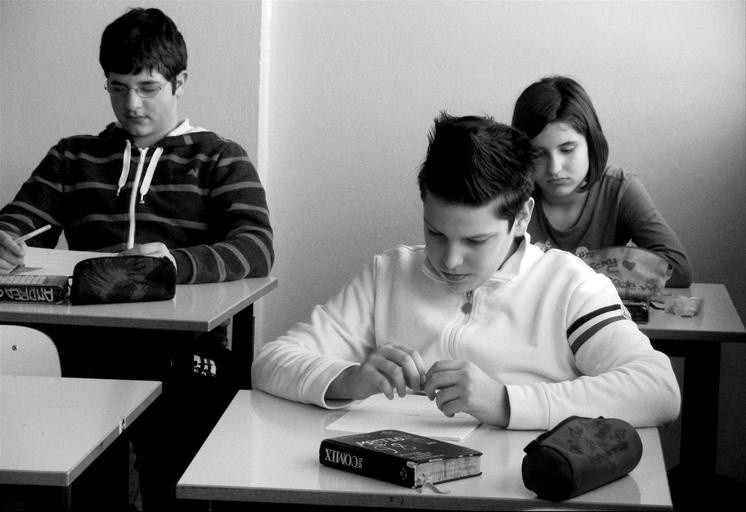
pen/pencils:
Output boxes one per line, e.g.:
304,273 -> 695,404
14,224 -> 52,246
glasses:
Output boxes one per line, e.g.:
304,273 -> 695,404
104,79 -> 169,98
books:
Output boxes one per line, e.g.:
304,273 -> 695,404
1,273 -> 68,303
318,429 -> 485,489
620,298 -> 651,326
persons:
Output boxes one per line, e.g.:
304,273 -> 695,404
510,76 -> 693,290
249,114 -> 685,432
1,8 -> 275,511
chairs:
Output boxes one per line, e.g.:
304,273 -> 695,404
1,325 -> 64,375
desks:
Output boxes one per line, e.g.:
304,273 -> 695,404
1,260 -> 280,412
608,282 -> 745,501
0,375 -> 161,511
173,386 -> 676,511
